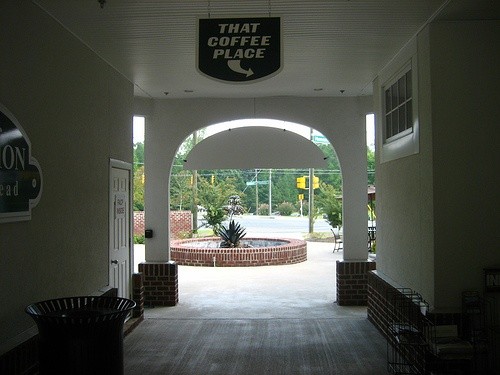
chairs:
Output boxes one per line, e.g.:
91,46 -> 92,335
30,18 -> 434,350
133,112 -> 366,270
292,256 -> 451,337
367,226 -> 376,254
330,228 -> 344,253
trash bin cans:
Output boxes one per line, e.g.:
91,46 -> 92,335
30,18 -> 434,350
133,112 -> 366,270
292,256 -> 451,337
24,296 -> 137,375
